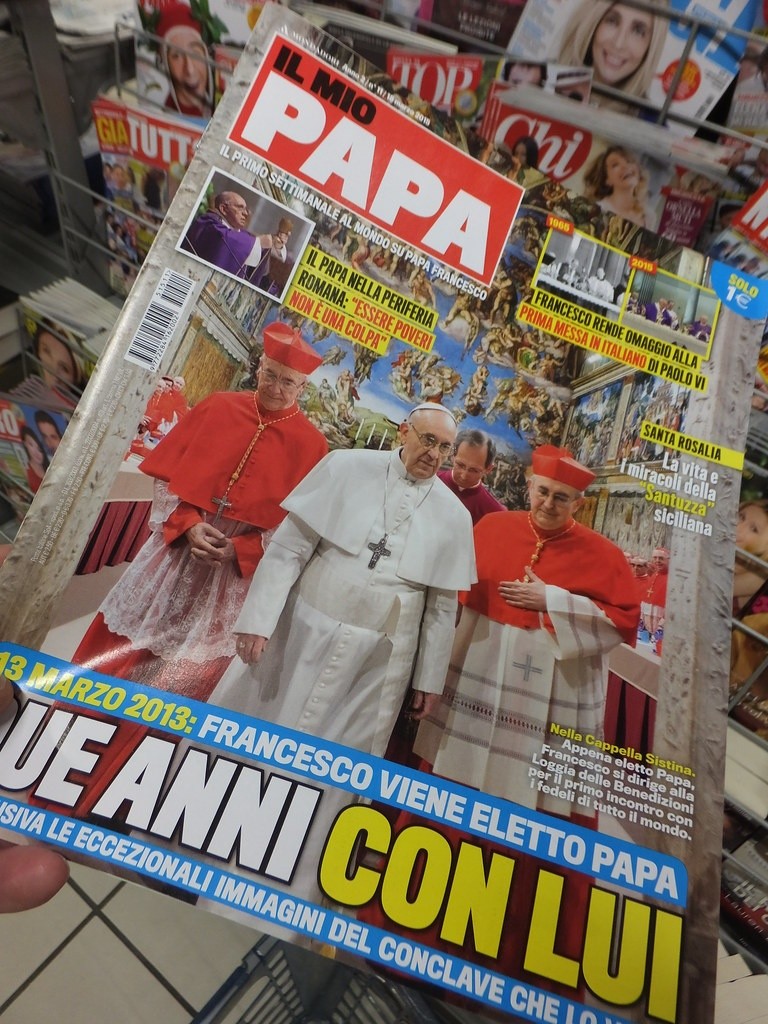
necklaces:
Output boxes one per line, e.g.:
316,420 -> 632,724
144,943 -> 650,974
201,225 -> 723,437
368,453 -> 437,569
646,573 -> 661,597
213,390 -> 300,531
525,511 -> 574,583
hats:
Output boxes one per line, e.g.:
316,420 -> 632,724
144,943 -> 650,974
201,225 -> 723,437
532,444 -> 597,492
155,0 -> 214,115
262,322 -> 324,375
408,402 -> 459,427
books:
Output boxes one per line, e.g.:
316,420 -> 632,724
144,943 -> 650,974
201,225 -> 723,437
0,0 -> 768,1024
279,0 -> 768,280
91,92 -> 211,295
0,373 -> 79,524
19,277 -> 123,388
133,0 -> 275,120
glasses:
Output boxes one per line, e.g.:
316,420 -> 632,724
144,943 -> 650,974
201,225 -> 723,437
258,369 -> 306,388
407,422 -> 457,457
452,459 -> 488,474
535,485 -> 578,504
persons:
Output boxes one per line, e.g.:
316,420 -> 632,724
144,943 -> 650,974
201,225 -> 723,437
155,7 -> 214,115
28,325 -> 670,1000
510,138 -> 539,168
101,161 -> 167,276
731,500 -> 768,697
502,59 -> 547,87
559,0 -> 669,127
34,410 -> 63,462
21,427 -> 51,492
186,192 -> 291,288
583,147 -> 654,228
34,318 -> 82,388
552,255 -> 615,303
629,291 -> 710,342
